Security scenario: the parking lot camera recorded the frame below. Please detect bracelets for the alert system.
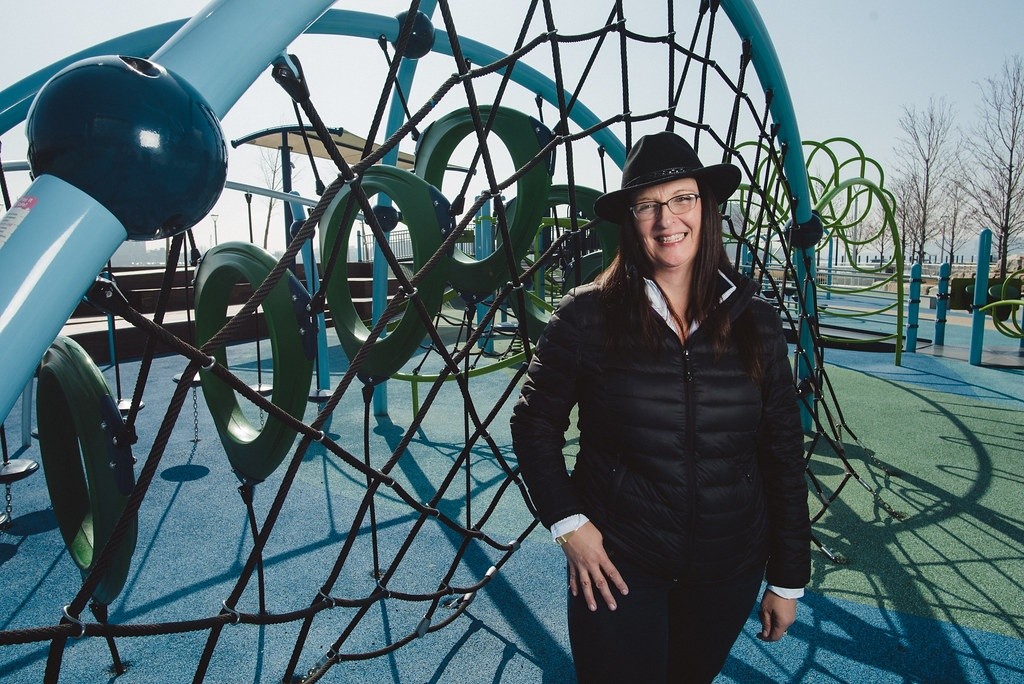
[556,531,576,546]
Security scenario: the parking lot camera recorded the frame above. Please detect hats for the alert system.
[592,131,742,225]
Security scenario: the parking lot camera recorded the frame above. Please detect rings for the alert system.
[783,630,787,634]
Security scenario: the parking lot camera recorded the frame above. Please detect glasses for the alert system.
[627,193,703,222]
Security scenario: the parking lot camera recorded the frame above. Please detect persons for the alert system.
[511,133,812,684]
